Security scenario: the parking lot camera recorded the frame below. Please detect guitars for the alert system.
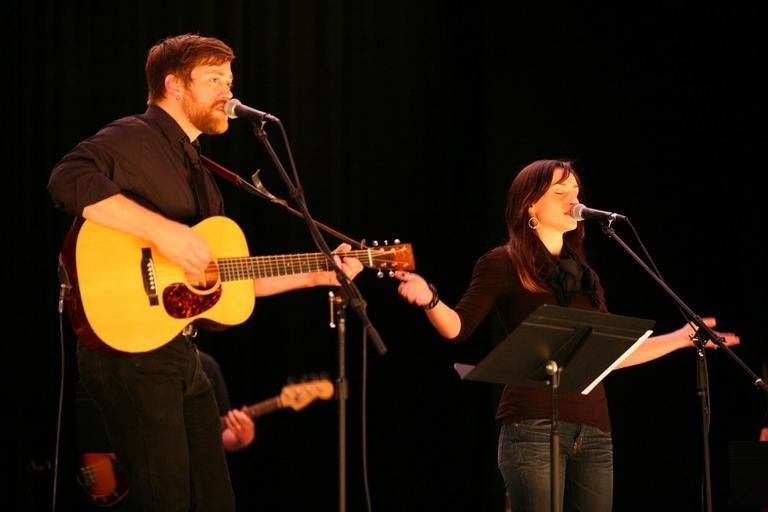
[59,215,416,358]
[66,372,335,509]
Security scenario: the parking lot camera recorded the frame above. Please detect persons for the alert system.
[394,159,739,512]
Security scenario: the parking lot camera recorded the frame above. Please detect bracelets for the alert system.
[417,283,439,310]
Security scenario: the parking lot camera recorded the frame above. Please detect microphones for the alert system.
[570,203,628,221]
[224,97,280,123]
